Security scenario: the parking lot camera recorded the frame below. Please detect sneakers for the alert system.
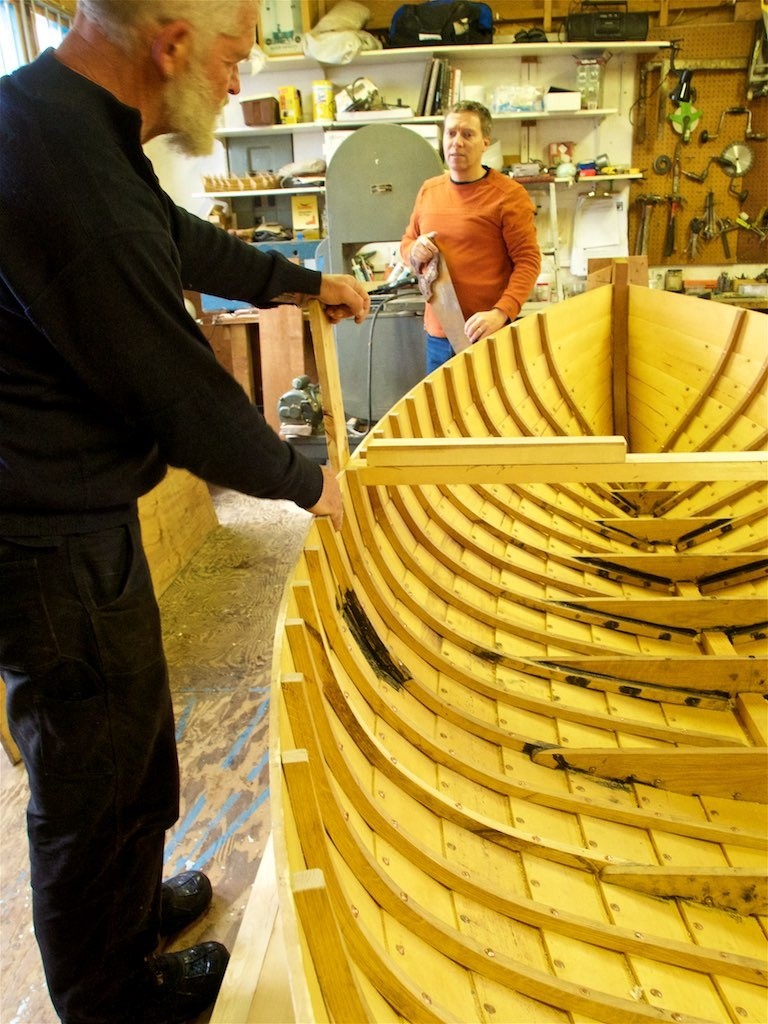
[158,869,213,941]
[119,941,231,1024]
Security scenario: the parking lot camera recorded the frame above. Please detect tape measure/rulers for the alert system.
[658,58,748,124]
[637,61,662,143]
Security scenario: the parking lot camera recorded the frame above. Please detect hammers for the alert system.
[664,194,687,257]
[632,194,660,256]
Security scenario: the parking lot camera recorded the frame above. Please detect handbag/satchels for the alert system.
[389,0,494,50]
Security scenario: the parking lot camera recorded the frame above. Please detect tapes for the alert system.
[577,159,595,169]
[597,154,609,167]
[654,155,671,174]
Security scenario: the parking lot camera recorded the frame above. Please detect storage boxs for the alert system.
[277,87,304,123]
[312,81,340,121]
[290,195,320,241]
[239,93,278,126]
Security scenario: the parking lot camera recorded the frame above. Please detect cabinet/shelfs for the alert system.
[204,38,672,321]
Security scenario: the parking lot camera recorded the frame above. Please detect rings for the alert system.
[477,328,484,334]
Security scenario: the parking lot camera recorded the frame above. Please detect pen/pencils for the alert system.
[368,289,384,295]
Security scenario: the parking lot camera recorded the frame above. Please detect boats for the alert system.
[267,257,767,1023]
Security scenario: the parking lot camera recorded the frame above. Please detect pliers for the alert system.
[671,143,682,179]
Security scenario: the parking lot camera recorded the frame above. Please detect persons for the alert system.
[400,99,542,374]
[0,0,369,1024]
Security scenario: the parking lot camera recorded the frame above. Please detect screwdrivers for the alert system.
[740,213,761,232]
[736,218,759,234]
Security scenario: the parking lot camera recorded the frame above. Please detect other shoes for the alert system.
[512,28,547,44]
